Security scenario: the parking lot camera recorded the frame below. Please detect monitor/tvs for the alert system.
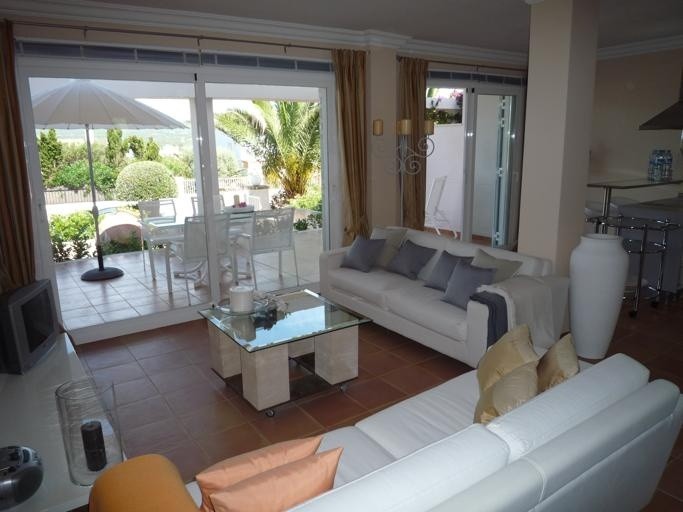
[0,279,58,375]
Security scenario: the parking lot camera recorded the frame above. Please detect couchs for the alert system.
[88,346,683,512]
[320,224,570,369]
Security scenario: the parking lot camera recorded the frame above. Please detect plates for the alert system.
[215,296,269,316]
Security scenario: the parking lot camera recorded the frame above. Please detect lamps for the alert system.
[373,118,435,225]
[636,66,683,142]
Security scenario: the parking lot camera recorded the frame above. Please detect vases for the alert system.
[568,230,629,360]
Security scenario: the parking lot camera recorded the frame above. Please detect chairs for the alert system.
[137,199,300,308]
[425,175,459,238]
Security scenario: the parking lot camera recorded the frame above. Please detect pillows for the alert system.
[339,233,522,311]
[470,325,580,425]
[194,436,322,511]
[209,446,344,511]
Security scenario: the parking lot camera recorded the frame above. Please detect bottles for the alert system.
[55,375,123,488]
[647,149,673,183]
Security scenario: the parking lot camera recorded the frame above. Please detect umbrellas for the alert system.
[30,80,190,280]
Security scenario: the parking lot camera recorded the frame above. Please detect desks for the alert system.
[1,332,127,512]
[588,215,681,319]
[587,178,683,234]
